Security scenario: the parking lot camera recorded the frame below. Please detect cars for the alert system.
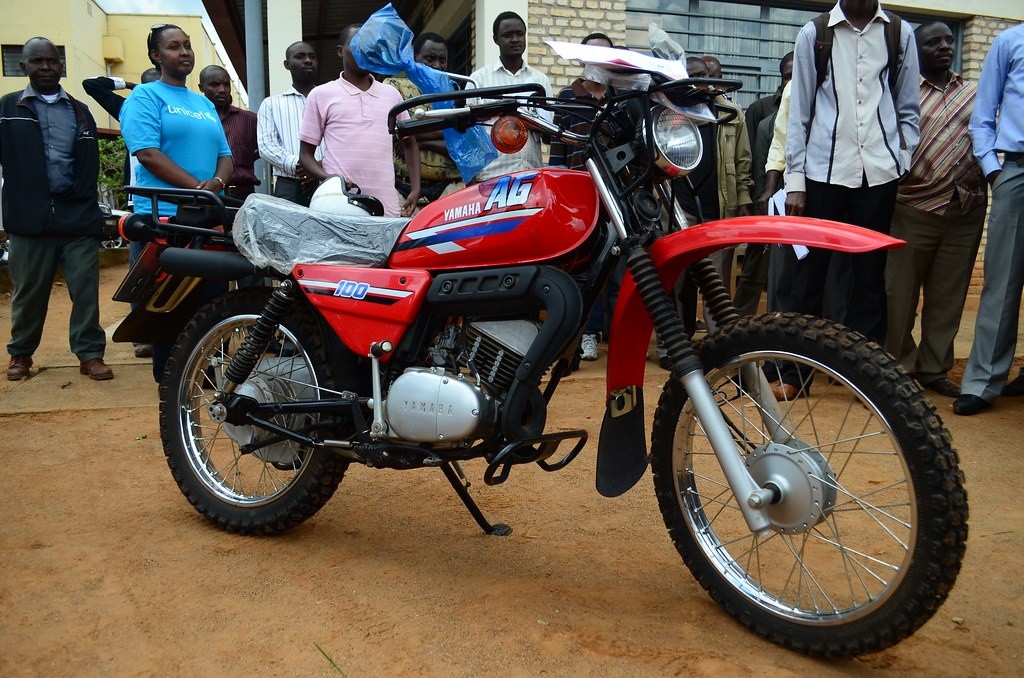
[99,201,133,249]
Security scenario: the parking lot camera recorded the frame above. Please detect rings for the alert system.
[304,176,307,179]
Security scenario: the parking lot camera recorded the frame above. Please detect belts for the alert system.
[1006,152,1024,161]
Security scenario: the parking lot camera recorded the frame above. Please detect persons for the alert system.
[465,12,554,182]
[257,24,465,225]
[549,33,651,362]
[684,0,1024,416]
[0,37,115,381]
[82,24,261,357]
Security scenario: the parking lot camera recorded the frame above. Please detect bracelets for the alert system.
[214,177,225,188]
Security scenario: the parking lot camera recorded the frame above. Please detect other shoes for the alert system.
[695,319,707,332]
[134,345,153,357]
[1000,375,1024,396]
[659,356,674,370]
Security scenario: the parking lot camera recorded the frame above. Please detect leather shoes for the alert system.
[771,381,810,401]
[953,394,996,415]
[922,378,961,397]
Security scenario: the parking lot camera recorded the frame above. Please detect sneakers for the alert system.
[579,334,598,360]
[80,358,113,380]
[7,356,33,380]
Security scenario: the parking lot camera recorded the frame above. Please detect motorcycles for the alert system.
[107,23,970,661]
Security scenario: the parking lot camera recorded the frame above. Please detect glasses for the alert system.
[150,24,178,45]
